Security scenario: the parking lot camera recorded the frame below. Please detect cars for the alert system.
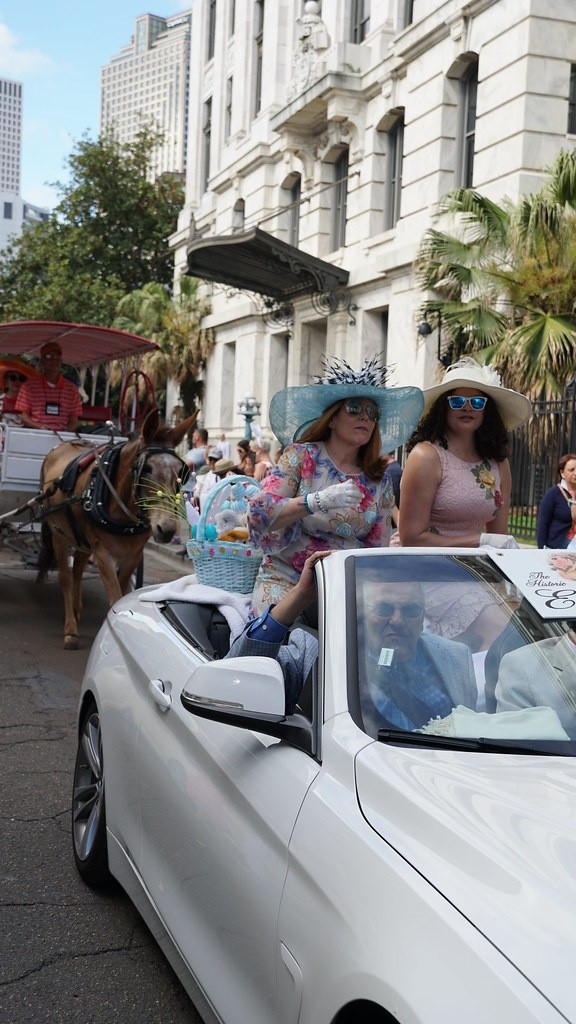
[72,549,576,1024]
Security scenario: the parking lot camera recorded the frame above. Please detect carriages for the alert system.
[0,321,201,651]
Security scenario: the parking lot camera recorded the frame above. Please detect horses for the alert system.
[35,403,200,651]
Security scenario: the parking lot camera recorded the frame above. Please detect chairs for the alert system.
[482,594,575,713]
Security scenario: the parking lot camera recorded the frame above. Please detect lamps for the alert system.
[418,309,454,373]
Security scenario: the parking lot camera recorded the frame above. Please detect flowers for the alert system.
[445,357,501,387]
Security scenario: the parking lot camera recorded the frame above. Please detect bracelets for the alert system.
[314,491,329,515]
[296,492,314,514]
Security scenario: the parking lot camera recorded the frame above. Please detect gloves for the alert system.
[480,533,521,549]
[304,478,365,514]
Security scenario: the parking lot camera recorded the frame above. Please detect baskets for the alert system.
[186,474,263,594]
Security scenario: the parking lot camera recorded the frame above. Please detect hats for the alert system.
[268,350,424,457]
[208,448,222,458]
[0,359,40,389]
[258,435,271,450]
[421,357,531,431]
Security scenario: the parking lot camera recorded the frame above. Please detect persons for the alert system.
[221,548,479,742]
[247,349,424,632]
[495,617,576,728]
[537,453,576,549]
[174,445,246,556]
[15,342,82,431]
[0,353,39,437]
[236,439,255,477]
[390,356,532,650]
[217,430,231,460]
[181,427,209,473]
[249,435,275,481]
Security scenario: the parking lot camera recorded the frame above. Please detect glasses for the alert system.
[366,602,423,618]
[238,449,242,452]
[447,396,488,410]
[44,354,62,359]
[9,374,27,383]
[345,400,381,422]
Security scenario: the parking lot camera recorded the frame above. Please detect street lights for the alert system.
[238,397,262,442]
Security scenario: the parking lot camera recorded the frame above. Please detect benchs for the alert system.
[0,397,112,449]
[164,600,229,657]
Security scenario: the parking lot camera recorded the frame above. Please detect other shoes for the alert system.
[175,550,187,556]
